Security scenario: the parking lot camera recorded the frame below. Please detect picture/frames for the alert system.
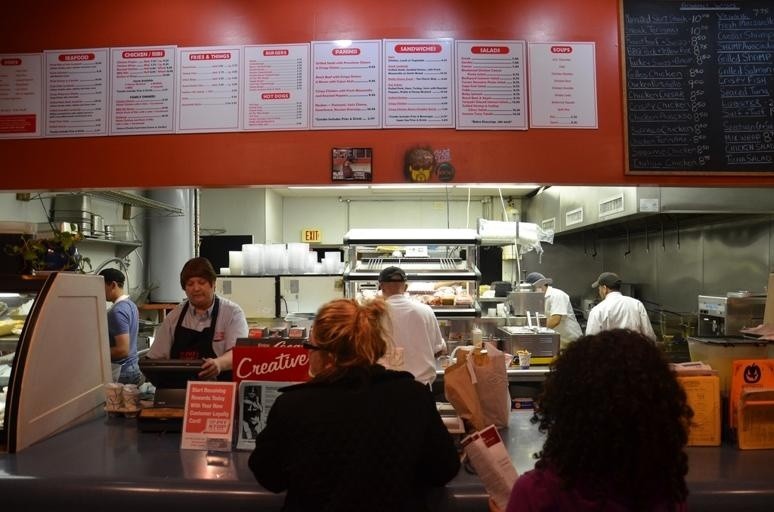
[331,148,372,181]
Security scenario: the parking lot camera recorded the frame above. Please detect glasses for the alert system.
[302,338,334,353]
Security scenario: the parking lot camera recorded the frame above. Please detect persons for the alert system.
[487,329,692,511]
[525,272,583,356]
[137,256,249,382]
[375,266,448,407]
[584,273,657,345]
[247,297,462,511]
[98,268,144,386]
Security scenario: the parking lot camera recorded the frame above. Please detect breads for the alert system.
[0,320,25,335]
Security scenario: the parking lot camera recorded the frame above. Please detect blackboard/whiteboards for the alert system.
[619,0,774,176]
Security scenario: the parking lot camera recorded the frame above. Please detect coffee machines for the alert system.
[697,294,767,338]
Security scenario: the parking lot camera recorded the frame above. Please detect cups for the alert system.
[496,304,504,316]
[518,352,530,368]
[487,308,496,317]
[105,383,122,409]
[663,335,673,352]
[122,384,139,408]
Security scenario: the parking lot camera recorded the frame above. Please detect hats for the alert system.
[99,268,125,283]
[180,257,216,291]
[591,272,622,289]
[526,272,546,284]
[378,266,407,290]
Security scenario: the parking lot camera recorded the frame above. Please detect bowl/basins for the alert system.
[227,243,341,275]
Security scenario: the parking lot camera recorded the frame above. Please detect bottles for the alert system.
[472,328,482,349]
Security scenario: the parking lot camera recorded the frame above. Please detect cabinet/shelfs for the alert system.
[343,227,482,318]
